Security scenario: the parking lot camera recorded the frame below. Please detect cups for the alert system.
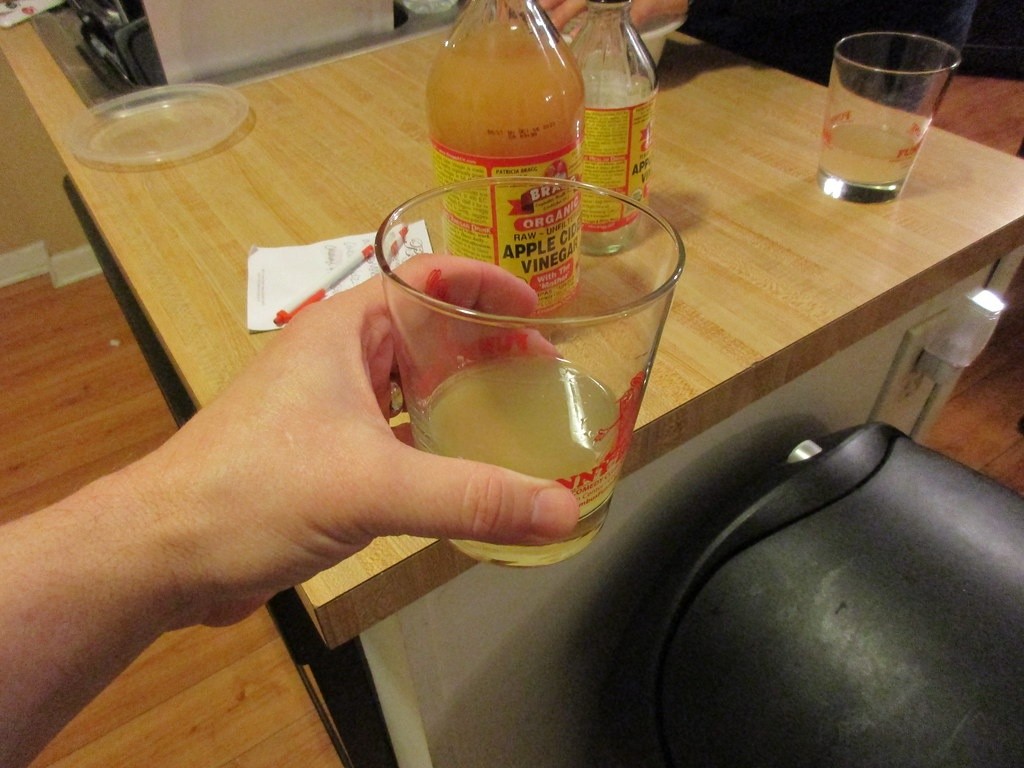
[816,31,962,205]
[374,173,685,569]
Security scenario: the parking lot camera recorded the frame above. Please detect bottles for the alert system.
[567,0,660,255]
[423,0,586,317]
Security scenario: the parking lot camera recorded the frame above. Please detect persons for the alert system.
[0,250,581,768]
[541,0,974,116]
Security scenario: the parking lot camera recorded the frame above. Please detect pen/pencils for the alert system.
[273,244,376,326]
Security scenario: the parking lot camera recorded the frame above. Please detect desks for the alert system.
[0,0,1023,767]
